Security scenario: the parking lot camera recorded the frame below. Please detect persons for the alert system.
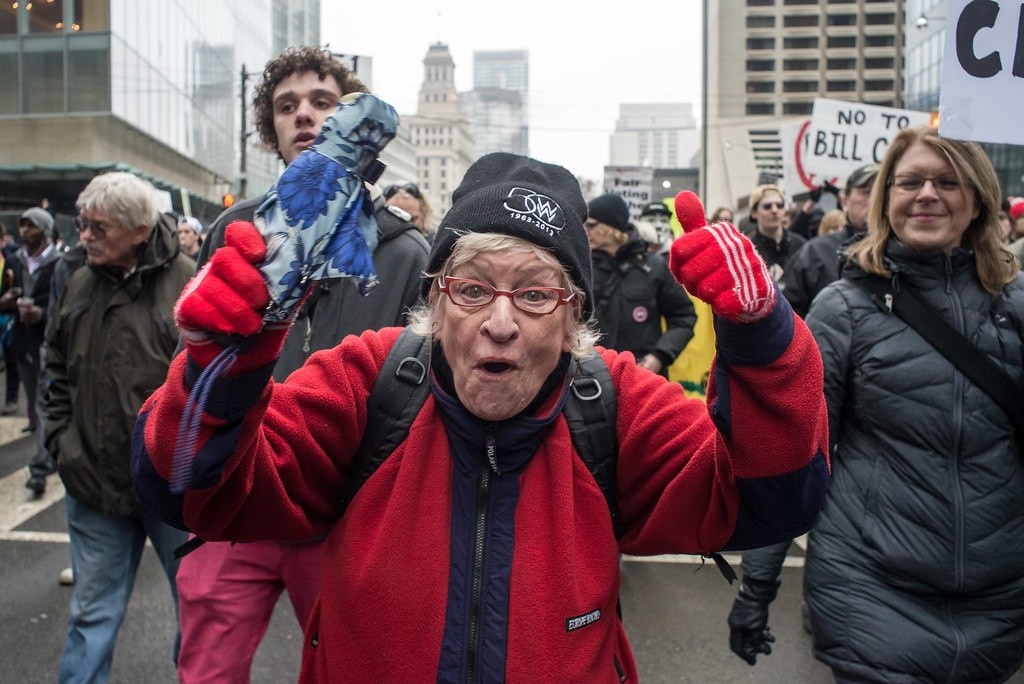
[0,207,88,492]
[782,180,846,240]
[42,198,54,217]
[43,171,197,684]
[709,207,733,224]
[178,216,204,261]
[583,194,697,380]
[130,152,832,684]
[746,184,807,270]
[384,183,435,245]
[173,46,432,684]
[777,165,879,320]
[996,195,1024,244]
[639,202,675,264]
[727,125,1024,684]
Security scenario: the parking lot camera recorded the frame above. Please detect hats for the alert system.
[748,184,786,223]
[19,207,54,239]
[844,165,879,196]
[588,194,633,232]
[639,203,673,220]
[421,153,595,325]
[176,214,205,247]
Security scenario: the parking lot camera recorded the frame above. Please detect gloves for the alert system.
[174,221,317,376]
[668,190,776,322]
[728,574,782,665]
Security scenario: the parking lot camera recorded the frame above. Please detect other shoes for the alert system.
[1,403,19,415]
[24,474,47,492]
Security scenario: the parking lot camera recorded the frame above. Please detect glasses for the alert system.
[585,220,600,230]
[756,200,784,210]
[382,183,424,202]
[887,175,973,192]
[438,276,576,314]
[75,217,123,239]
